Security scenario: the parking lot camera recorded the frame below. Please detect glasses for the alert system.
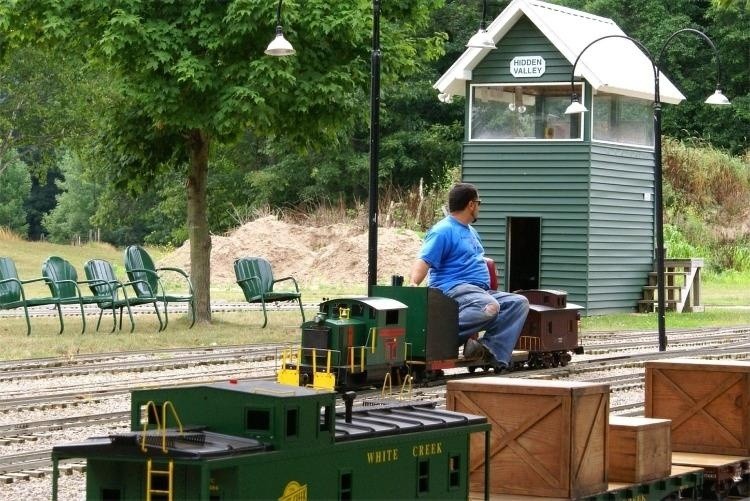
[472,200,481,206]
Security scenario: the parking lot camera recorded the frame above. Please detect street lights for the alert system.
[263,0,381,291]
[564,26,734,353]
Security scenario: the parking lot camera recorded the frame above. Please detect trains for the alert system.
[278,282,583,394]
[45,355,747,499]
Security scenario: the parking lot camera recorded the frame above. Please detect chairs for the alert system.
[234,256,305,328]
[0,245,196,336]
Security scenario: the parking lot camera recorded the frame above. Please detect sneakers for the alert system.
[462,337,491,361]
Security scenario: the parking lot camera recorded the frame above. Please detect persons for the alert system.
[406,184,529,368]
